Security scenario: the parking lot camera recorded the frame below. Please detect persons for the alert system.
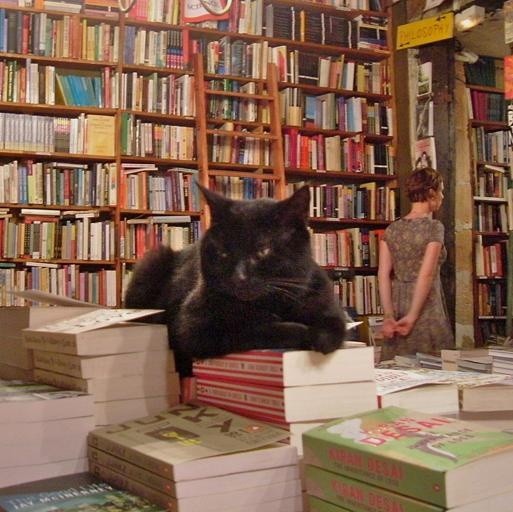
[378,169,447,357]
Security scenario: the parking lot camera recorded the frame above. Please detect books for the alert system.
[0,0,397,322]
[414,58,513,318]
[0,307,513,512]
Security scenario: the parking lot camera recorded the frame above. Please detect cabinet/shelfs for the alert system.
[0,0,405,327]
[462,54,512,348]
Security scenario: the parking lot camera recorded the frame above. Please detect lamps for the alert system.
[453,3,485,32]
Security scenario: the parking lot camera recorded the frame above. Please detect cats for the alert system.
[123,178,347,381]
